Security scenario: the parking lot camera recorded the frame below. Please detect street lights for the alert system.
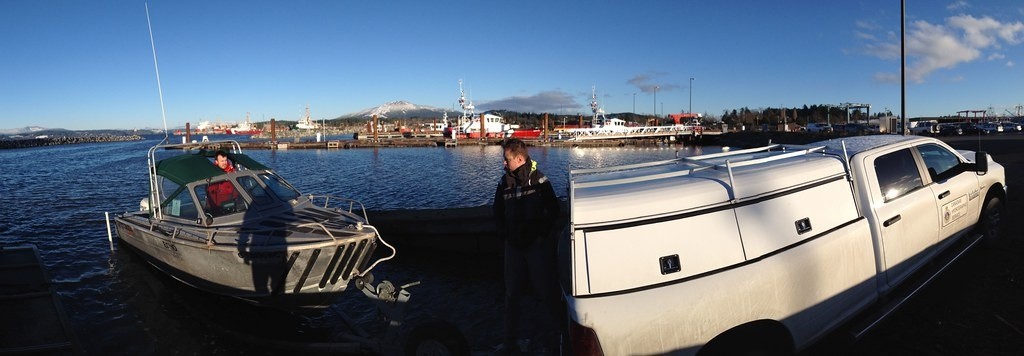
[654,86,659,126]
[633,93,637,127]
[690,78,695,126]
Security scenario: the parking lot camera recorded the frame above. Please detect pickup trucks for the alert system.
[567,135,1010,356]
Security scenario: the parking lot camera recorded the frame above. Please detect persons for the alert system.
[486,139,561,356]
[206,151,239,218]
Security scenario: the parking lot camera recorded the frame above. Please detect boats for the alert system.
[115,140,380,304]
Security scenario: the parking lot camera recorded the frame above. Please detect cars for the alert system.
[806,122,1022,135]
